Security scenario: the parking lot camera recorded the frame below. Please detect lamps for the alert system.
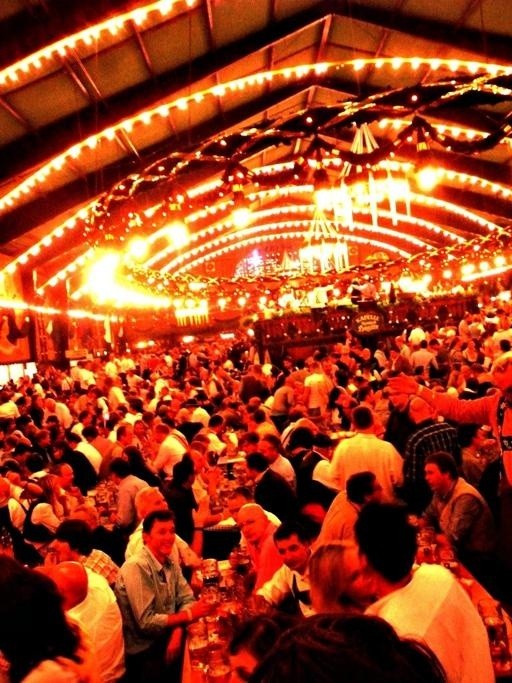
[299,206,348,274]
[341,125,411,229]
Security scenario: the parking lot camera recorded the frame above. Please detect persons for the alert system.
[0,272,512,683]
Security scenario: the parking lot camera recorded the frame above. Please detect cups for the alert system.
[415,527,512,601]
[187,559,236,683]
[96,482,120,525]
[212,470,246,515]
